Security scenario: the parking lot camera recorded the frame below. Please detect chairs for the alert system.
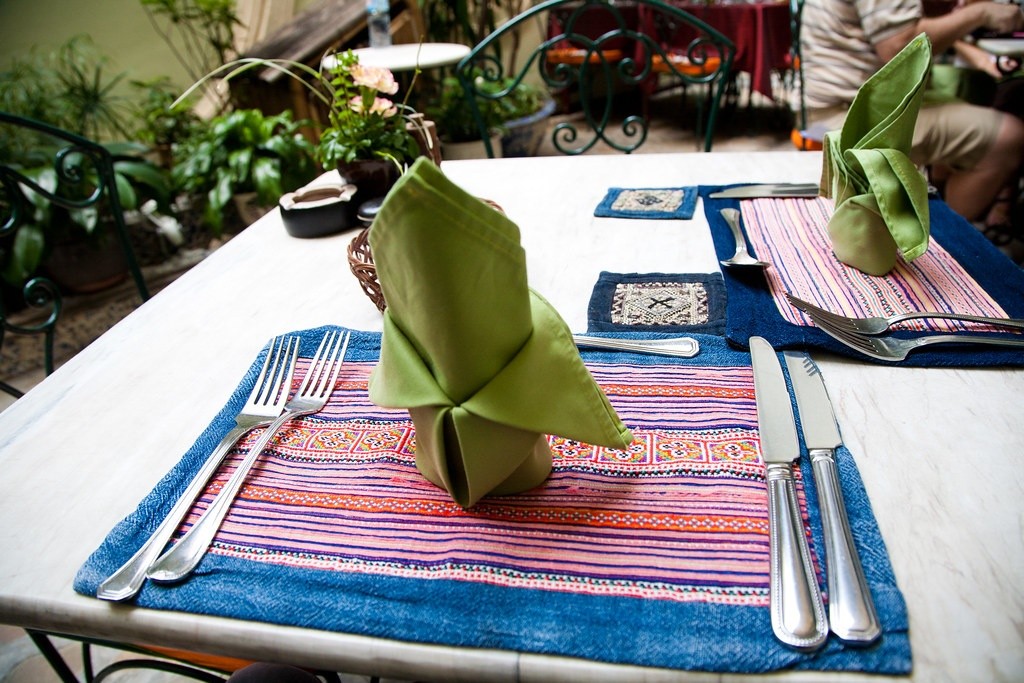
[790,0,828,151]
[452,0,739,159]
[1,111,344,683]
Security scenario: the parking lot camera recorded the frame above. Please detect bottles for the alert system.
[366,0,391,50]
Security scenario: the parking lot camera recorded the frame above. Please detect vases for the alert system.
[336,145,401,195]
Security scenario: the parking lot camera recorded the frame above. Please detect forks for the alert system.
[144,330,354,582]
[783,290,1024,332]
[95,332,300,602]
[804,306,1024,363]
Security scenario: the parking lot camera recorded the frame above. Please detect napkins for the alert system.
[369,155,634,508]
[819,31,934,277]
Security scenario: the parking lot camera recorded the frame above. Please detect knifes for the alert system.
[782,350,882,647]
[709,183,821,199]
[750,334,828,648]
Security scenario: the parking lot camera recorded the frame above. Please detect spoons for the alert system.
[719,207,772,272]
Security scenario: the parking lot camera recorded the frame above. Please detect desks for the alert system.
[549,0,793,109]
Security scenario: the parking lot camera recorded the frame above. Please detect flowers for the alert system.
[169,47,432,177]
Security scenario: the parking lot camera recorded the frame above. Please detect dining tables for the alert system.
[978,38,1024,57]
[324,43,475,75]
[0,149,1024,683]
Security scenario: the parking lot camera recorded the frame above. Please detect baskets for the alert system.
[346,196,507,316]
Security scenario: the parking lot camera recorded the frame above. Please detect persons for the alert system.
[800,0,1024,234]
